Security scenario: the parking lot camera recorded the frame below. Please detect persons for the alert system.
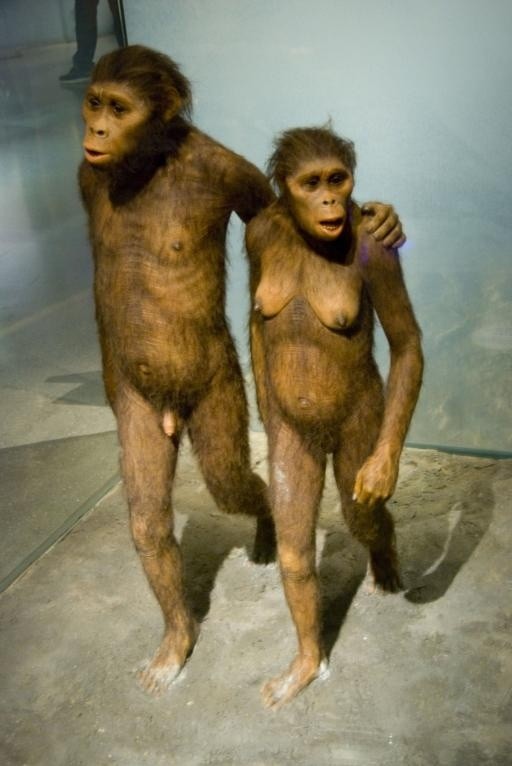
[59,2,124,83]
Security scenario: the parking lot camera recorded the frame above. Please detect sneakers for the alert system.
[58,65,93,84]
